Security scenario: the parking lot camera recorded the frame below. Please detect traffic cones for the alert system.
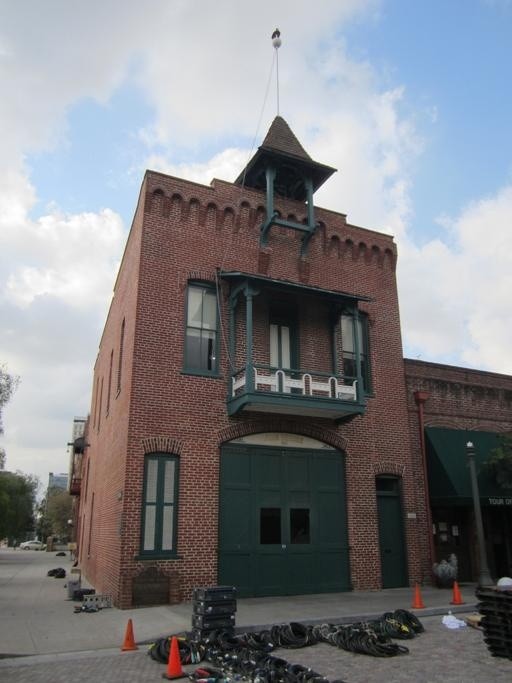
[448,579,467,605]
[119,616,144,651]
[161,634,189,681]
[409,581,426,609]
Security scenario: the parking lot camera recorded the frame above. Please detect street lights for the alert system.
[463,438,499,602]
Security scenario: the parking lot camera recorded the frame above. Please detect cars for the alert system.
[19,539,47,551]
[54,535,71,545]
